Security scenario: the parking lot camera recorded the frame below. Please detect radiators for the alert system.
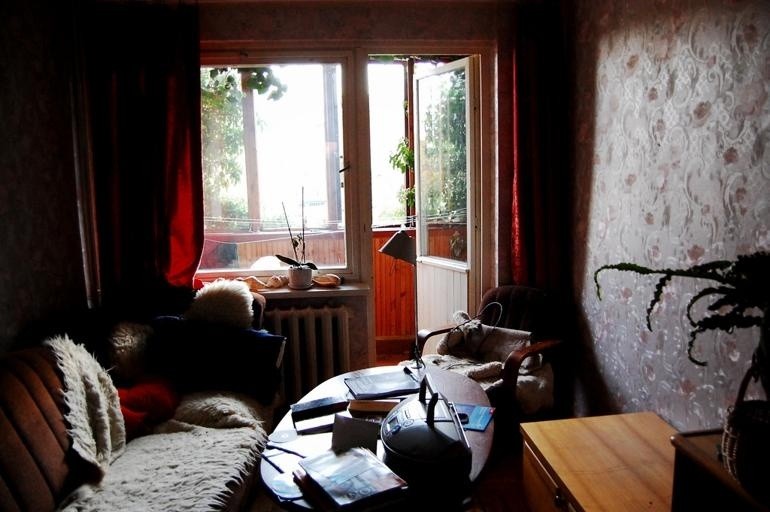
[264,307,353,405]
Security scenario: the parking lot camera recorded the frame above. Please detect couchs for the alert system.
[0,316,287,512]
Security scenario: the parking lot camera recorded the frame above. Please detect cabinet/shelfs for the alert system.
[520,411,770,512]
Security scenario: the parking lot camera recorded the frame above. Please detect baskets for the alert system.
[721,353,769,497]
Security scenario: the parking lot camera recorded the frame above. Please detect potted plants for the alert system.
[276,233,317,290]
[594,251,770,496]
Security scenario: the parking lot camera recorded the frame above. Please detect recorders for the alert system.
[379,373,473,484]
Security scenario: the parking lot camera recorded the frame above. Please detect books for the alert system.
[290,369,496,512]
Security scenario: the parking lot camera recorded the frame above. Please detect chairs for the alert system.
[420,285,576,452]
